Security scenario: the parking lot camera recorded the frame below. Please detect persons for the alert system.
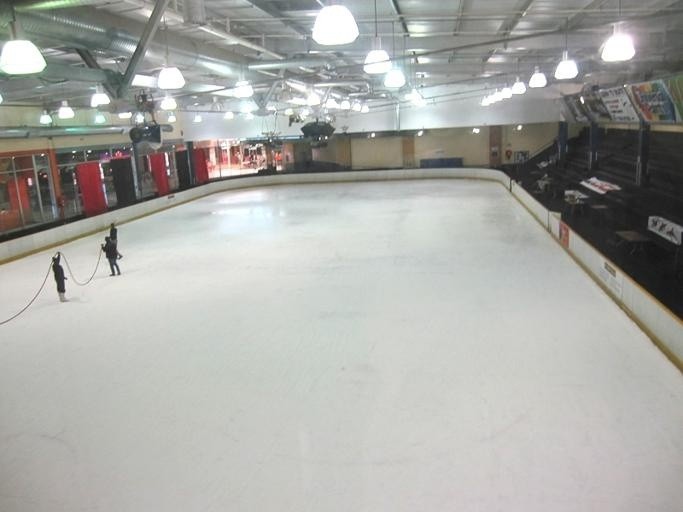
[100,236,121,277]
[51,251,69,302]
[108,222,123,259]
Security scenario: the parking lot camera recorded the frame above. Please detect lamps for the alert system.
[527,48,548,89]
[86,93,374,127]
[0,1,47,76]
[553,17,578,80]
[599,3,636,63]
[56,80,76,121]
[382,20,405,90]
[363,1,392,75]
[480,58,527,107]
[405,51,427,112]
[154,3,186,91]
[39,97,54,126]
[312,3,361,48]
